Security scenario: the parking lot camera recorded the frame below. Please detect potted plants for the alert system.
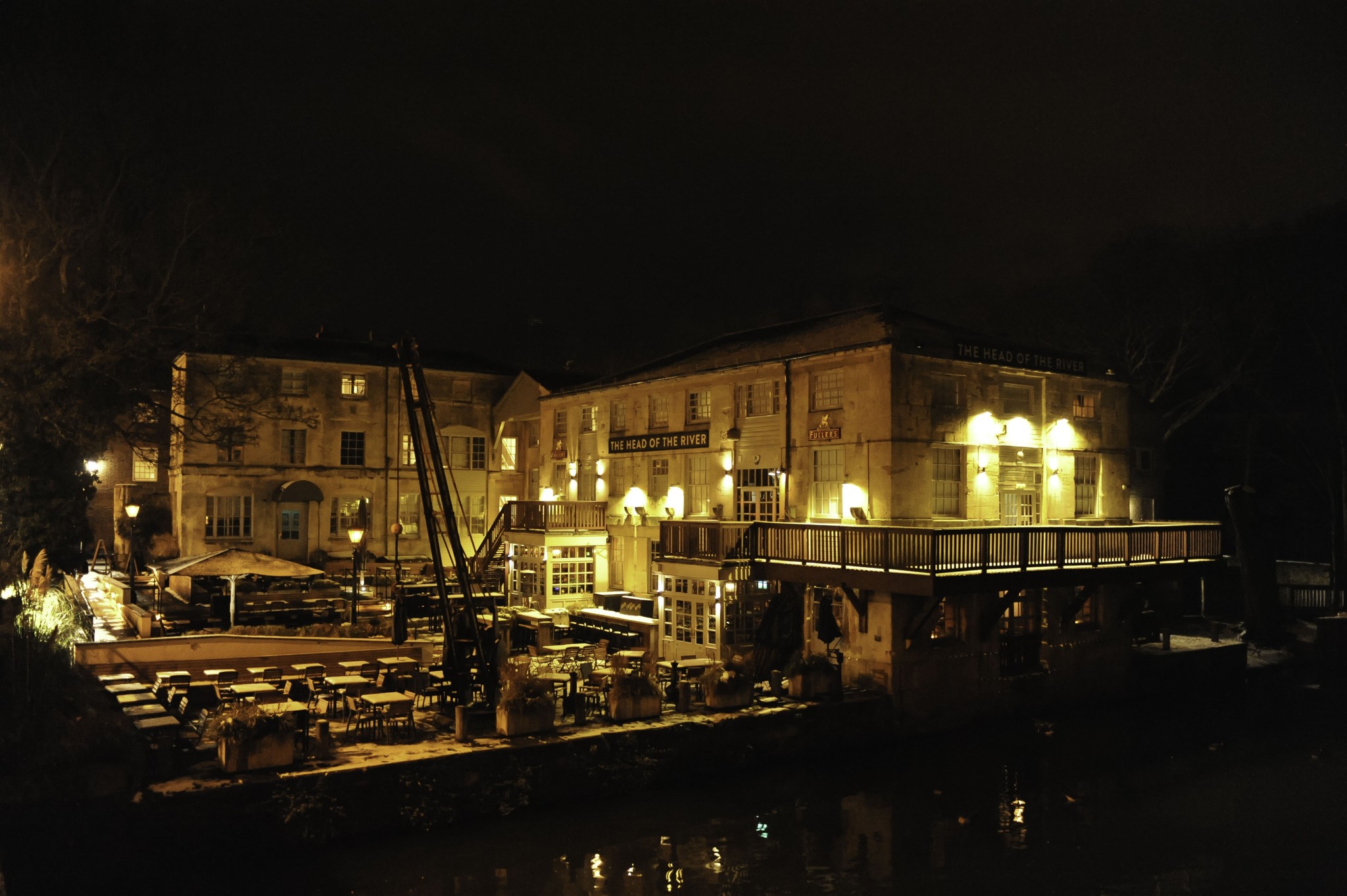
[787,651,840,697]
[699,661,755,707]
[209,698,298,773]
[608,652,663,720]
[496,661,556,735]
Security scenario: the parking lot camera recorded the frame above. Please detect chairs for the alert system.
[513,614,703,709]
[218,668,310,751]
[152,675,208,757]
[252,601,285,625]
[299,661,485,745]
[313,599,346,624]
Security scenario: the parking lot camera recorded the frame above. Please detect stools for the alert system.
[555,625,569,643]
[517,623,537,652]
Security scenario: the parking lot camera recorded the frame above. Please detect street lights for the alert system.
[124,497,140,604]
[347,522,364,625]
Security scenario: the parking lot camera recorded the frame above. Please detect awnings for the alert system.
[272,480,324,501]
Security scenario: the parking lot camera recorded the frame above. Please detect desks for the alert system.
[203,667,308,714]
[396,583,507,631]
[291,657,418,741]
[429,669,478,712]
[378,567,410,576]
[515,613,553,630]
[98,671,191,757]
[534,643,723,700]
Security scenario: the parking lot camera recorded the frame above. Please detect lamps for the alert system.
[624,505,676,517]
[850,507,866,520]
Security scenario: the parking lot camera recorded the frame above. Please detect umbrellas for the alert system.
[356,496,368,572]
[145,547,326,627]
[392,585,407,660]
